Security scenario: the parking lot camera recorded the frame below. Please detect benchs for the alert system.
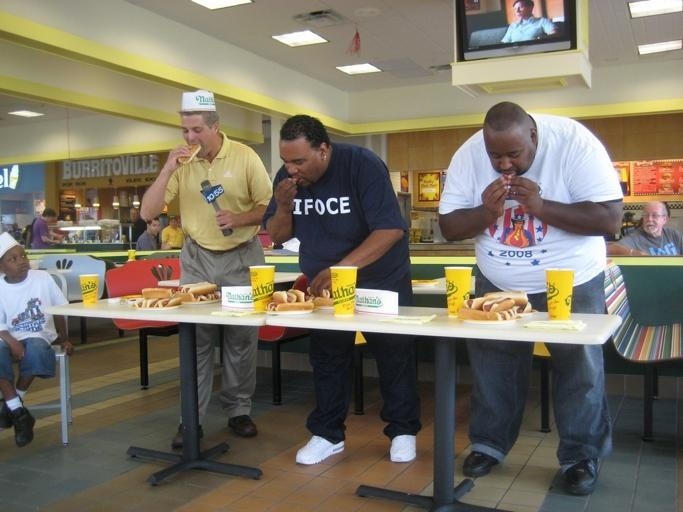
[354,274,555,434]
[107,258,321,405]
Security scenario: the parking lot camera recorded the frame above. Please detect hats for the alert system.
[0,229,20,259]
[180,88,218,113]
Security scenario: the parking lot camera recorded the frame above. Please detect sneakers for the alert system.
[565,455,600,497]
[0,397,37,449]
[170,424,205,450]
[225,414,258,438]
[389,433,417,463]
[295,434,345,466]
[462,449,500,478]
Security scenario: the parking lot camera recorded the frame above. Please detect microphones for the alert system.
[199,179,234,237]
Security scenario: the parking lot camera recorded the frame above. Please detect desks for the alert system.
[38,282,625,510]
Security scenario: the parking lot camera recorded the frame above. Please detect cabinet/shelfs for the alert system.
[45,219,126,248]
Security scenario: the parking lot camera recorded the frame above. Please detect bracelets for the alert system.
[629,249,635,256]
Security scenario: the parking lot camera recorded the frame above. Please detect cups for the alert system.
[545,270,575,321]
[248,265,275,314]
[128,249,136,260]
[79,273,99,307]
[329,265,360,317]
[443,266,473,320]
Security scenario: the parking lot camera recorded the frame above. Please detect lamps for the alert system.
[110,188,120,211]
[72,198,81,208]
[131,185,142,210]
[91,188,100,207]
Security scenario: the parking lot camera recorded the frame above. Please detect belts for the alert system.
[188,236,257,254]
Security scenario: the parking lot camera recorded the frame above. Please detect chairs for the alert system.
[41,255,124,343]
[13,272,73,444]
[603,263,683,440]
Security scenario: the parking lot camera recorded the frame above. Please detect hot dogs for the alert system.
[133,281,221,309]
[177,144,201,164]
[265,286,330,312]
[458,290,532,322]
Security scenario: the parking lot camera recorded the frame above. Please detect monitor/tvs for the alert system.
[455,0,579,62]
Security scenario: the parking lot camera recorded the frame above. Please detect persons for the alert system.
[500,0,562,46]
[22,219,38,248]
[263,116,422,465]
[127,209,184,254]
[139,90,273,447]
[436,102,623,497]
[606,201,682,262]
[34,208,63,249]
[0,231,74,448]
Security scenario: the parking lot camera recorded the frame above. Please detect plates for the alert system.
[463,310,539,325]
[263,308,314,315]
[134,300,183,311]
[313,305,335,311]
[178,298,222,305]
